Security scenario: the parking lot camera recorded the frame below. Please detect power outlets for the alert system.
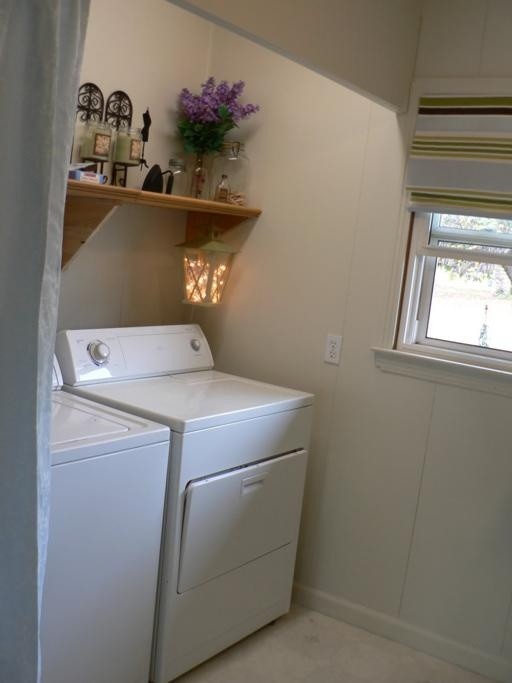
[324,333,342,365]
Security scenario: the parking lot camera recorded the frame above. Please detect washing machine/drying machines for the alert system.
[55,323,317,683]
[39,355,171,683]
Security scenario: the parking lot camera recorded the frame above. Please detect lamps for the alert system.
[176,224,237,308]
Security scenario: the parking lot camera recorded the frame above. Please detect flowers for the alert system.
[180,76,260,131]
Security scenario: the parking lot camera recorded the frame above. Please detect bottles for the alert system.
[214,173,232,203]
[80,119,113,160]
[115,125,143,159]
[208,140,249,208]
[163,158,188,195]
